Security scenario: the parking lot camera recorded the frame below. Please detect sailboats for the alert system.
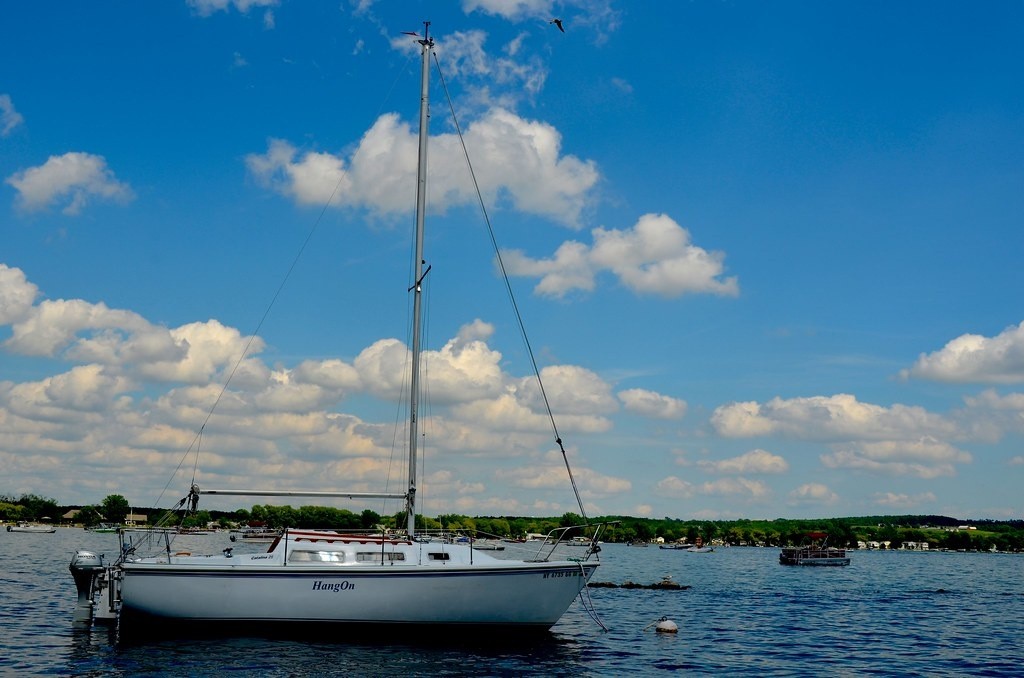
[70,18,603,629]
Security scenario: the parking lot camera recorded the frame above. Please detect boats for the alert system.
[780,532,852,567]
[740,540,747,548]
[234,539,275,543]
[509,535,527,544]
[526,533,554,545]
[83,523,122,534]
[467,537,505,551]
[242,528,280,539]
[659,539,693,550]
[649,575,689,590]
[566,536,604,546]
[7,520,56,533]
[687,545,713,553]
[626,541,648,547]
